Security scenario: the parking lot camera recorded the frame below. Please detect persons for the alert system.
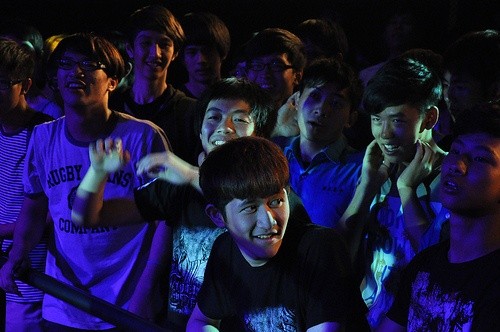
[108,5,200,165]
[0,36,56,332]
[246,28,307,107]
[298,9,449,149]
[0,22,71,120]
[71,77,312,332]
[375,101,500,332]
[187,136,370,332]
[0,32,173,332]
[335,55,451,332]
[171,13,229,100]
[437,30,500,153]
[284,58,366,229]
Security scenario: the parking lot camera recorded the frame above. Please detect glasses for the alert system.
[248,60,297,72]
[56,57,107,71]
[0,76,22,90]
